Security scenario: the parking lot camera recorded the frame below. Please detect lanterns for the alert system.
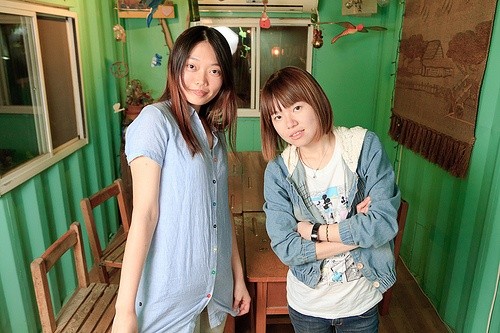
[272,44,287,60]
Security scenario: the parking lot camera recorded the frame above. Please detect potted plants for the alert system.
[126,80,151,113]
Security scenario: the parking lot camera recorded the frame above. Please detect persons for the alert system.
[110,25,251,333]
[259,66,402,333]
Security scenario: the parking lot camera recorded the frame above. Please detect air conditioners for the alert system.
[198,1,318,14]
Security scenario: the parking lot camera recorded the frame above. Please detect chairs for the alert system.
[81,178,130,283]
[31,221,120,332]
[380,196,409,315]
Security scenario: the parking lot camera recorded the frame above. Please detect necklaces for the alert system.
[301,134,331,179]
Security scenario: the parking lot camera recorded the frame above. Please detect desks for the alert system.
[223,152,290,332]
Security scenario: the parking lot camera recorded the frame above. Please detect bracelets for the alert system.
[325,223,329,241]
[310,222,322,243]
